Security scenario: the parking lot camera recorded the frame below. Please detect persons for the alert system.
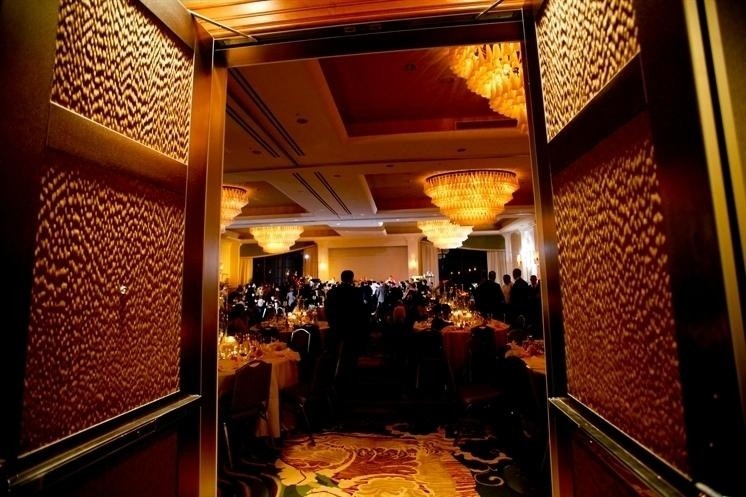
[225,269,542,389]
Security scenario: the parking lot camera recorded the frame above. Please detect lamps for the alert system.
[420,170,520,226]
[416,220,474,249]
[219,185,248,233]
[249,223,305,254]
[450,41,529,135]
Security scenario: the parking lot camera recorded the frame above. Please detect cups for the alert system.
[220,332,268,362]
